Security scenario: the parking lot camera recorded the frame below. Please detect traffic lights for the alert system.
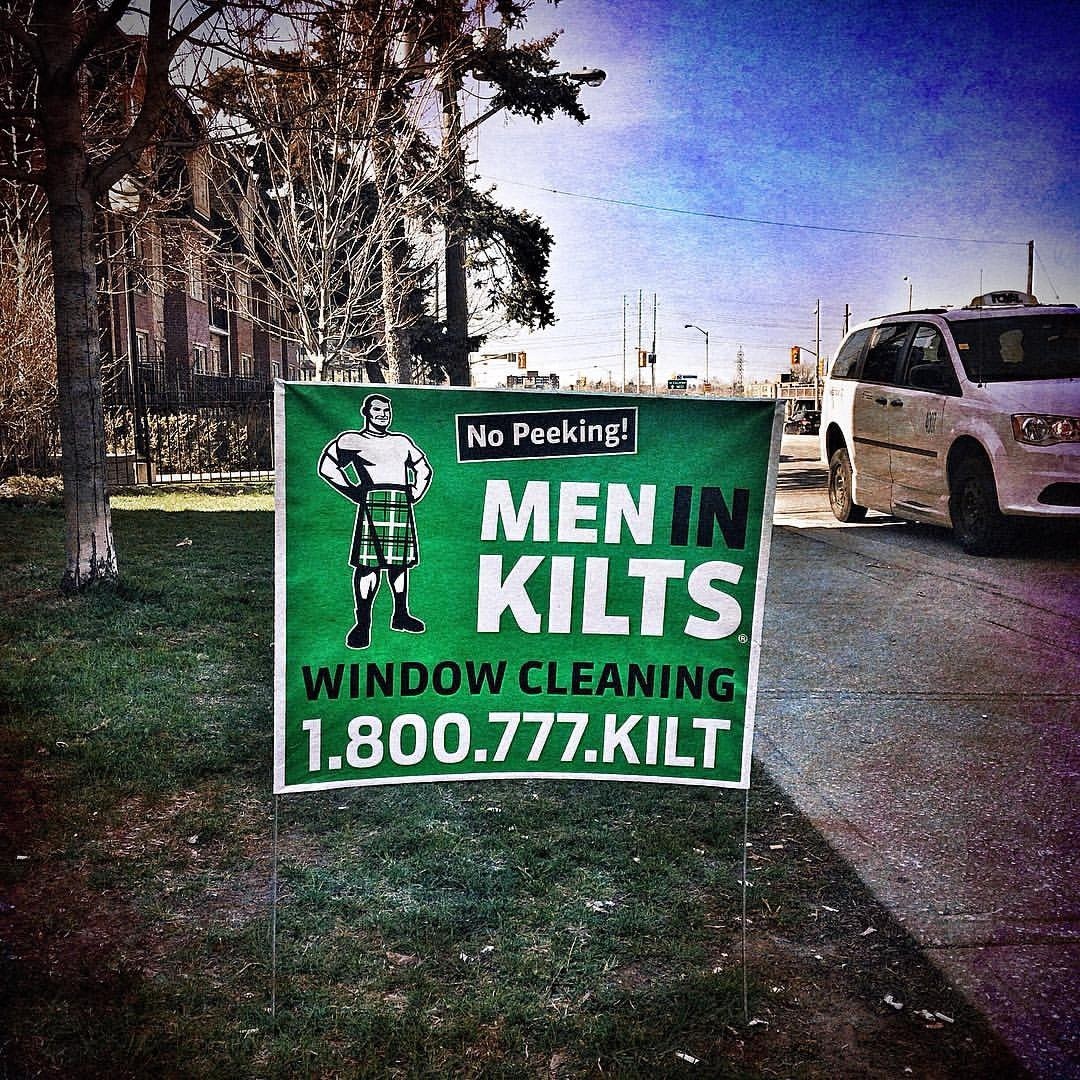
[792,348,802,366]
[818,358,824,376]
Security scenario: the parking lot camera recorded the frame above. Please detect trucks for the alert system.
[772,382,825,435]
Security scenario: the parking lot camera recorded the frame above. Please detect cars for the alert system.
[815,290,1080,558]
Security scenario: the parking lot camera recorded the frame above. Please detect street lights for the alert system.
[594,366,613,394]
[685,324,710,399]
